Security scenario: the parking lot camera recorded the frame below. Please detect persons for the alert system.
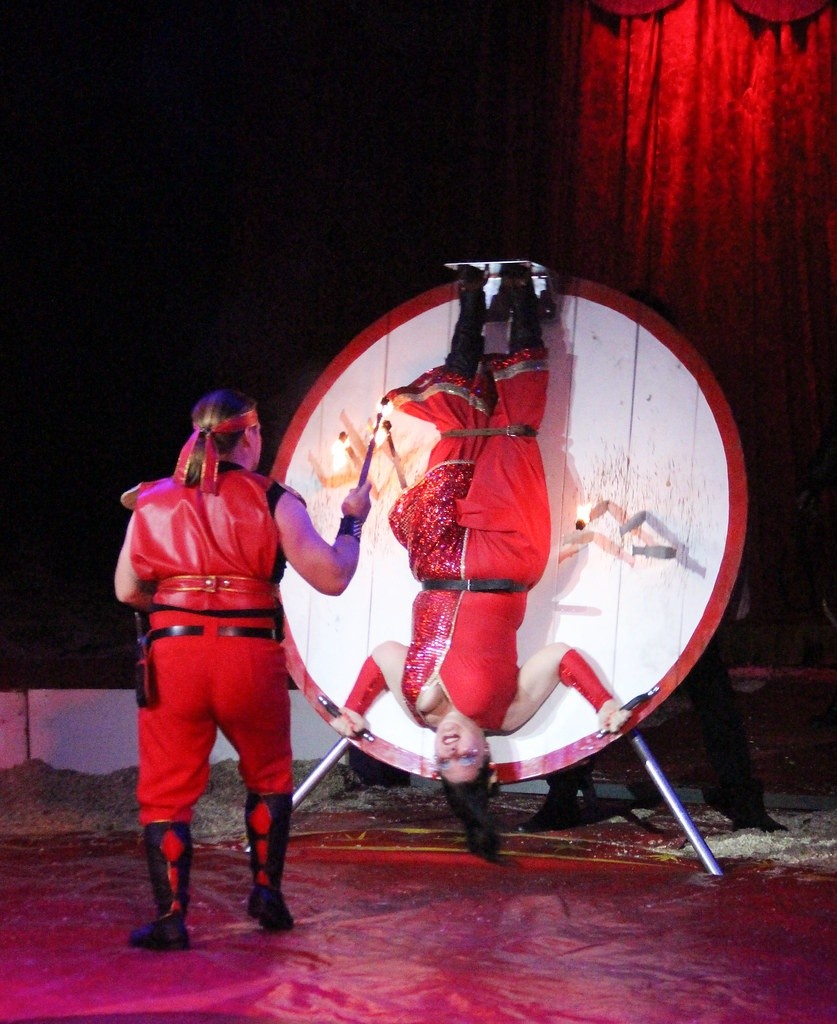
[329,264,633,810]
[115,388,373,950]
[795,361,837,731]
[515,287,788,834]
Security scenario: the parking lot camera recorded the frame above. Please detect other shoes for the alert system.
[129,923,191,952]
[247,888,293,930]
[809,705,837,728]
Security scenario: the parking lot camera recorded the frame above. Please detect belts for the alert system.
[423,578,525,592]
[145,625,281,640]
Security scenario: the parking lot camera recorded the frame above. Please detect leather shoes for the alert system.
[514,789,598,832]
[733,814,789,834]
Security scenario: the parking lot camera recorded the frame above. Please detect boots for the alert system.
[497,266,542,357]
[443,265,488,379]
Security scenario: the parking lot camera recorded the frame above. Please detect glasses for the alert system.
[438,753,477,770]
